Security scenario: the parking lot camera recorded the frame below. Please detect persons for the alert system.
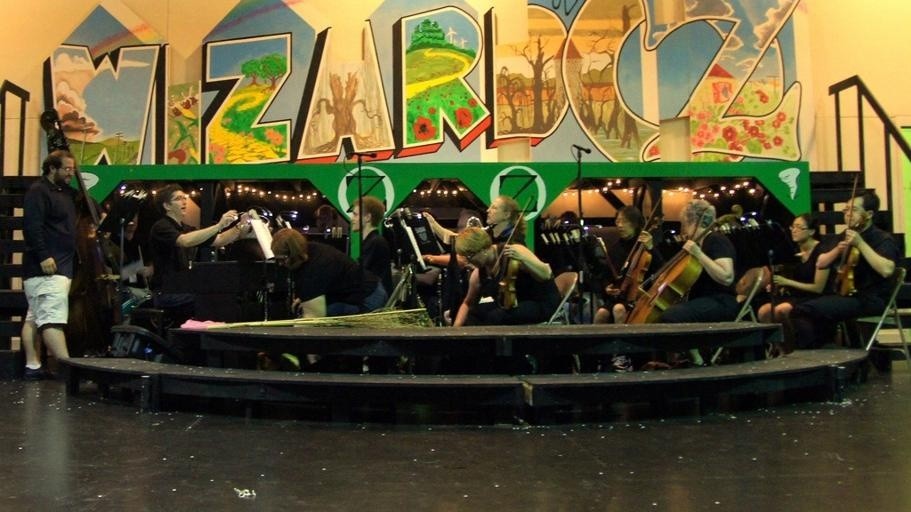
[20,151,78,379]
[592,186,903,353]
[149,186,562,327]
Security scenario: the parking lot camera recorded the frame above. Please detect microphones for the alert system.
[573,144,590,153]
[349,152,376,157]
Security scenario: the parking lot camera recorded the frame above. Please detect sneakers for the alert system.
[611,354,633,373]
[22,366,53,379]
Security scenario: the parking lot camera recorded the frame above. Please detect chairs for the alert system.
[855,266,910,359]
[543,270,578,325]
[708,268,770,365]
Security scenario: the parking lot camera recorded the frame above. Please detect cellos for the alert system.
[626,204,744,325]
[40,108,122,337]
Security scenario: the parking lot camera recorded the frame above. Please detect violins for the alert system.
[497,214,519,309]
[832,212,868,297]
[626,216,662,303]
